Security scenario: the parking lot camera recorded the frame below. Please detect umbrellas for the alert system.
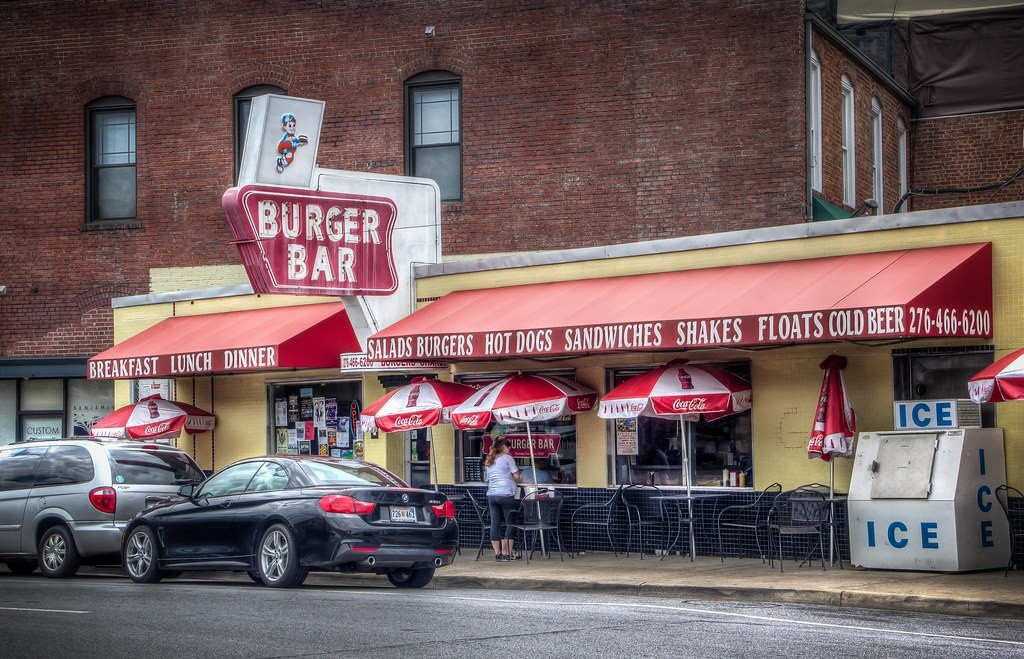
[452,371,599,556]
[968,348,1024,404]
[360,377,485,490]
[808,355,855,566]
[596,359,753,560]
[89,396,216,443]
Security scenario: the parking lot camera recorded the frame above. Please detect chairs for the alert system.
[448,482,842,572]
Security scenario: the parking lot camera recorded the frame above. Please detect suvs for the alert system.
[0,437,211,580]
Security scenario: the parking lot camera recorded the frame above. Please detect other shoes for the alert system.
[495,552,503,560]
[502,553,524,560]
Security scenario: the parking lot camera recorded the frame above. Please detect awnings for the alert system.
[86,301,362,379]
[365,241,994,362]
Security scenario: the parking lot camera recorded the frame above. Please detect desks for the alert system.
[787,496,846,571]
[648,494,731,559]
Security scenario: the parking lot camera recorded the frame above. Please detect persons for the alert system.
[521,458,554,483]
[485,439,522,561]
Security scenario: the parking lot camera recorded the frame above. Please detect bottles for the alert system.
[723,440,749,487]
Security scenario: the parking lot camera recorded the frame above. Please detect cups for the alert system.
[548,485,556,498]
[514,485,537,499]
[645,473,654,486]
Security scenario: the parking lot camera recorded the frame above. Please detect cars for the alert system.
[120,454,459,588]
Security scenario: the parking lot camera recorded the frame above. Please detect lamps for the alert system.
[424,26,435,38]
